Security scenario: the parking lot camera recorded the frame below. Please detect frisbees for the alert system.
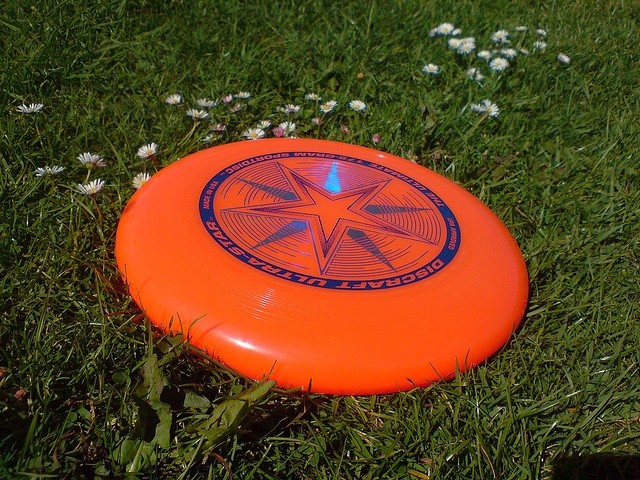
[115,138,529,396]
